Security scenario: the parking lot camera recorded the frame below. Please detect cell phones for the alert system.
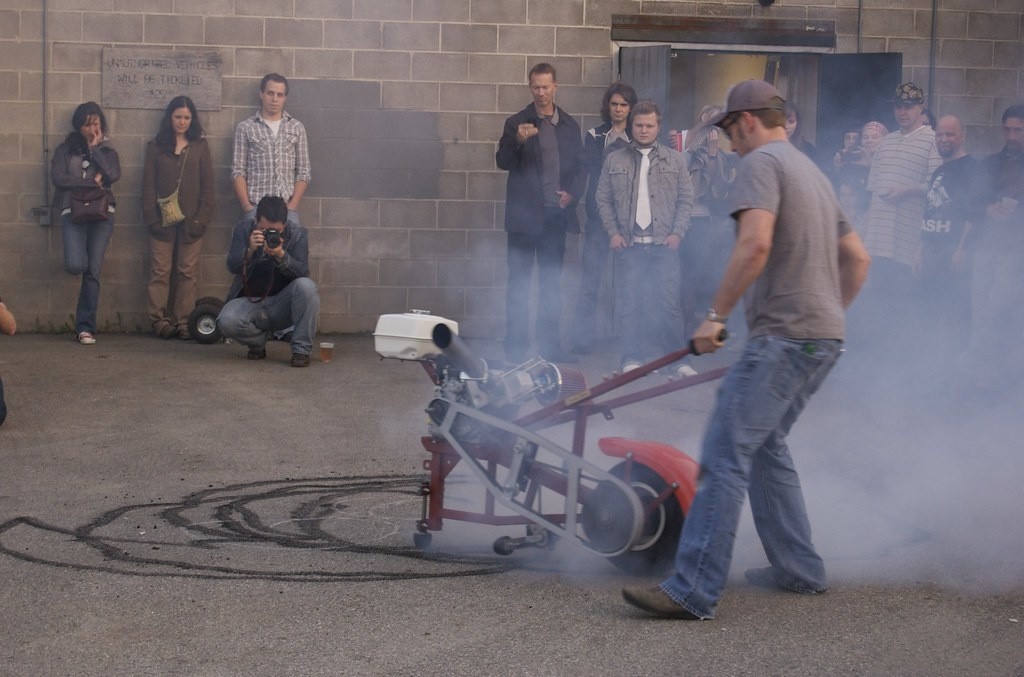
[527,116,537,128]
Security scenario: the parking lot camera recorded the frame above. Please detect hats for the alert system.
[704,79,785,126]
[888,83,924,104]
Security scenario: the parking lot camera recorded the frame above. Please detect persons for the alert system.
[144,95,214,340]
[0,298,17,426]
[621,76,869,619]
[496,63,587,365]
[569,83,1024,408]
[51,102,121,344]
[217,194,321,367]
[232,73,311,341]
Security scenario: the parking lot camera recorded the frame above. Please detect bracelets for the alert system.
[242,201,249,209]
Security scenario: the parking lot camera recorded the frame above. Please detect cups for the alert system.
[320,342,334,362]
[1003,197,1017,214]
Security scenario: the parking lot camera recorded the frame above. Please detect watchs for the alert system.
[707,307,726,324]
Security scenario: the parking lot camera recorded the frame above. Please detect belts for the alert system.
[633,236,653,244]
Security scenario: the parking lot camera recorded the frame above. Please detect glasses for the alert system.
[722,111,755,140]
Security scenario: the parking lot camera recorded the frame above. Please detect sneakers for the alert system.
[160,324,176,340]
[291,353,309,367]
[621,585,699,620]
[176,322,194,340]
[78,331,96,344]
[744,565,796,592]
[248,347,266,359]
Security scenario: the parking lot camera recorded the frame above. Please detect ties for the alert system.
[636,148,653,231]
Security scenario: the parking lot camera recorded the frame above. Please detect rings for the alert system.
[525,129,529,135]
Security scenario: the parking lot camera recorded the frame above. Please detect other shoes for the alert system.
[668,365,697,379]
[507,350,531,363]
[540,349,578,363]
[282,331,295,341]
[622,364,639,374]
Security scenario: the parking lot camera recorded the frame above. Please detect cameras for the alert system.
[261,227,284,249]
[841,147,864,161]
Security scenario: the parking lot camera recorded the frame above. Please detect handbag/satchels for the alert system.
[157,189,186,228]
[70,188,109,222]
